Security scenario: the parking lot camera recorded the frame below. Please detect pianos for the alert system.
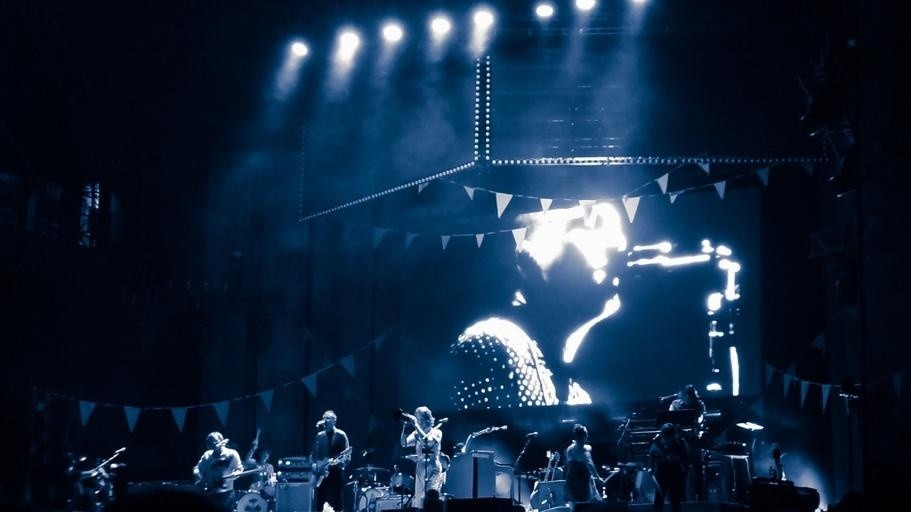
[441,451,515,499]
[625,427,703,446]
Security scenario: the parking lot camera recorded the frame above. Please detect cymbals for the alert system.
[356,468,389,473]
[401,455,418,463]
[737,422,764,430]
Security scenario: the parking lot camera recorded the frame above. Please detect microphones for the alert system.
[562,419,575,423]
[664,393,678,399]
[547,488,554,505]
[528,432,538,436]
[317,419,326,425]
[438,417,449,422]
[628,239,672,256]
[216,438,229,447]
[115,447,126,453]
[492,425,508,431]
[256,504,261,511]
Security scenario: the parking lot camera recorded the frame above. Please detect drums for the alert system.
[390,474,415,493]
[719,455,749,502]
[349,481,390,512]
[230,489,270,512]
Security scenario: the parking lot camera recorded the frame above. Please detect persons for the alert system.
[441,201,633,414]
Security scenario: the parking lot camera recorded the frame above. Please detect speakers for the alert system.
[475,450,496,498]
[275,482,314,512]
[531,479,567,512]
[440,454,474,499]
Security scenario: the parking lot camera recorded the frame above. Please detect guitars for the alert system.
[310,447,352,488]
[263,472,278,496]
[197,464,267,489]
[530,451,554,509]
